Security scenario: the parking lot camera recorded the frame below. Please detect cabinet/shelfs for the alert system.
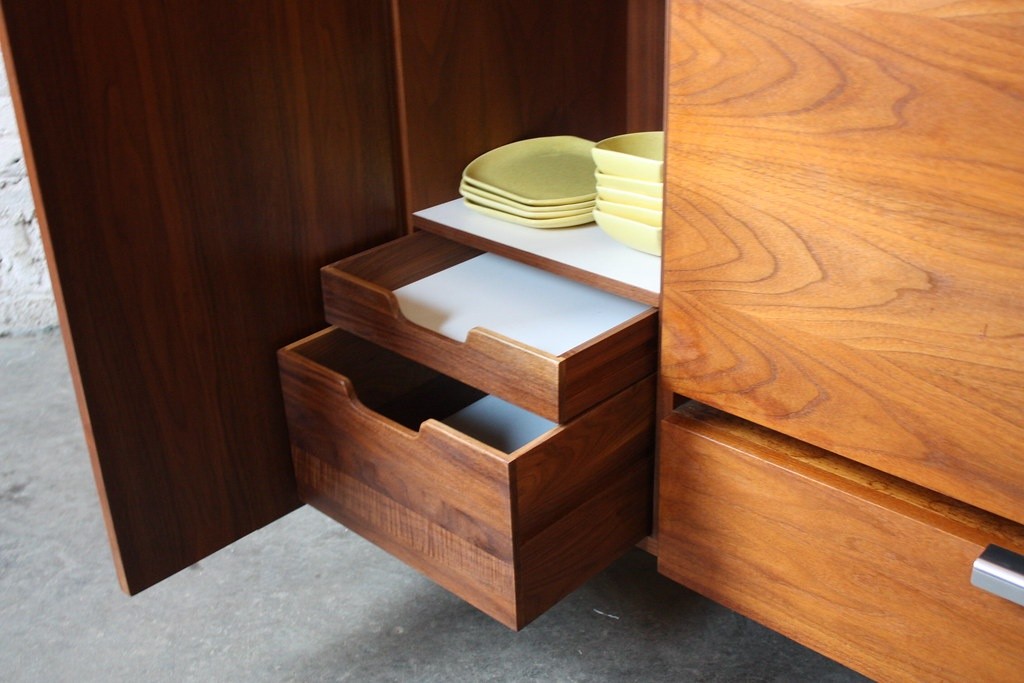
[0,0,1024,683]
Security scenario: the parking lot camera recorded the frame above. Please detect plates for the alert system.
[591,132,664,258]
[459,134,596,228]
